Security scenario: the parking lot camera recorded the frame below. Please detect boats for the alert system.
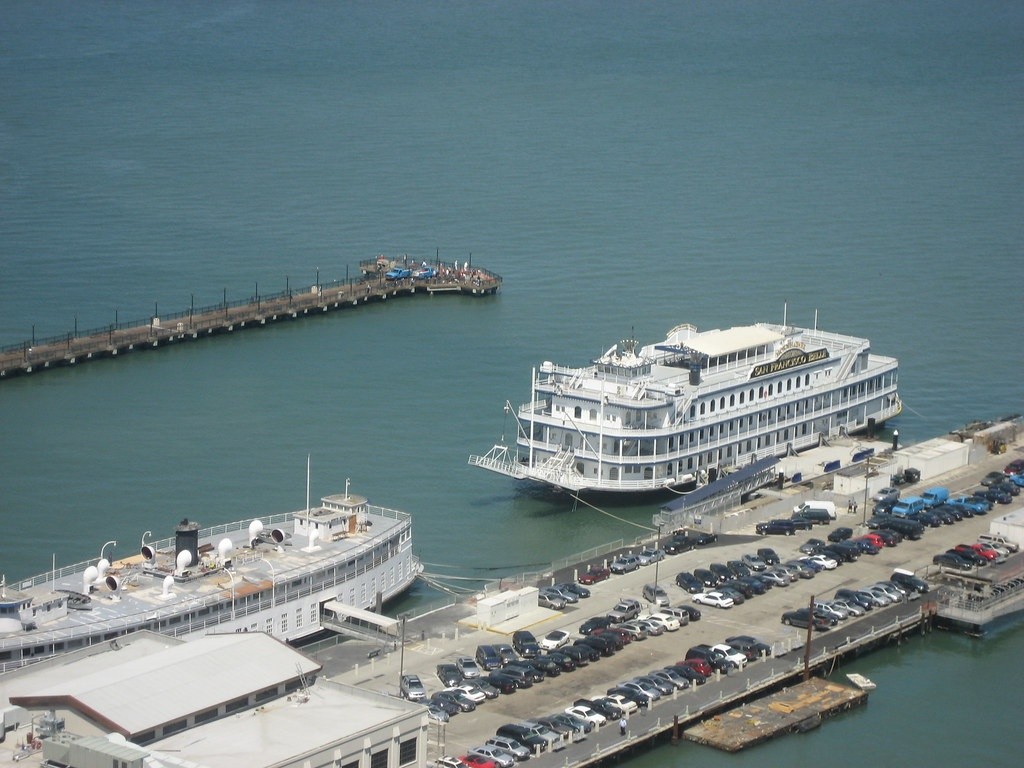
[0,489,425,678]
[474,320,902,506]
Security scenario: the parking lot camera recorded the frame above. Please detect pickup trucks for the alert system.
[412,265,435,279]
[386,266,412,280]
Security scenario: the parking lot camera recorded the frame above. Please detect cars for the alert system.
[635,551,663,565]
[547,585,579,603]
[399,673,422,699]
[698,532,716,546]
[577,568,609,582]
[678,458,1023,634]
[664,535,695,554]
[430,599,773,768]
[537,590,563,609]
[609,556,637,571]
[641,581,670,605]
[559,581,591,595]
